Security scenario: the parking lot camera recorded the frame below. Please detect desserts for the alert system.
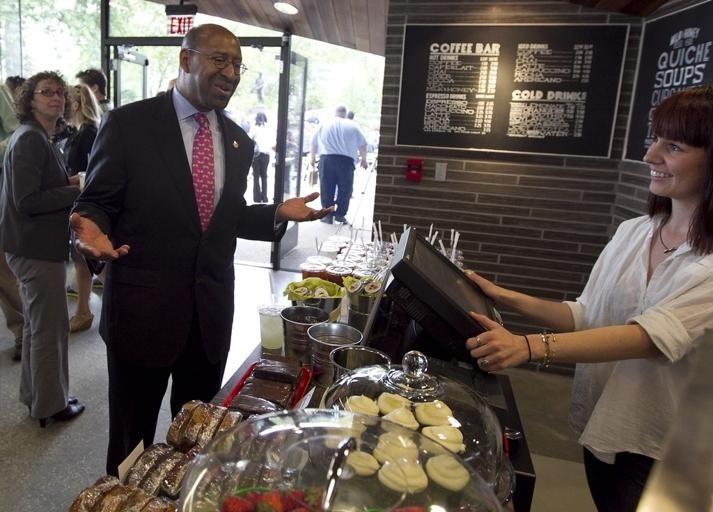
[325,391,469,493]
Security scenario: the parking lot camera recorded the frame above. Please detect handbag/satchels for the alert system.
[309,165,317,185]
[253,135,260,159]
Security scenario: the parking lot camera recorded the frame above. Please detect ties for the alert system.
[191,112,215,230]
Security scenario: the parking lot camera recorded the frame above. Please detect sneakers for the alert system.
[91,277,104,288]
[66,282,78,298]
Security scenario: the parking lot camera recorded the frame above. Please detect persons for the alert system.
[272,117,295,196]
[0,65,113,362]
[68,22,336,482]
[346,111,353,121]
[245,111,272,203]
[2,71,85,430]
[309,106,367,225]
[464,81,712,512]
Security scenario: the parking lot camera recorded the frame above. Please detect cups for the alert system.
[78,171,86,191]
[280,306,390,388]
[257,306,285,350]
[299,233,394,285]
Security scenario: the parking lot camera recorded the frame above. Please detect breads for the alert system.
[68,399,304,512]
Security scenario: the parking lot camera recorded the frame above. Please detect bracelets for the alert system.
[522,335,532,362]
[537,332,556,369]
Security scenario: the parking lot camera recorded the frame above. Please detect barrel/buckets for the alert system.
[279,278,391,386]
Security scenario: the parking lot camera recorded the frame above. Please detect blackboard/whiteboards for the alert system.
[621,0,713,165]
[395,23,631,159]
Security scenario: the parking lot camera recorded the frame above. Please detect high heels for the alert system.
[19,396,79,417]
[69,311,94,332]
[39,404,85,428]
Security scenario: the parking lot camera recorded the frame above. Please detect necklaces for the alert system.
[656,219,679,254]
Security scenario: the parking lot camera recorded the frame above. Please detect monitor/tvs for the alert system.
[385,225,499,339]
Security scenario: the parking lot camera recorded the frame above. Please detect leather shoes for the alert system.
[334,215,349,224]
[11,338,22,361]
[321,216,334,225]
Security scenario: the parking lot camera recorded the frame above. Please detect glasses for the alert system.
[31,88,66,98]
[187,48,248,76]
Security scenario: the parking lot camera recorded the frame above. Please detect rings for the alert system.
[479,359,488,369]
[475,335,480,347]
